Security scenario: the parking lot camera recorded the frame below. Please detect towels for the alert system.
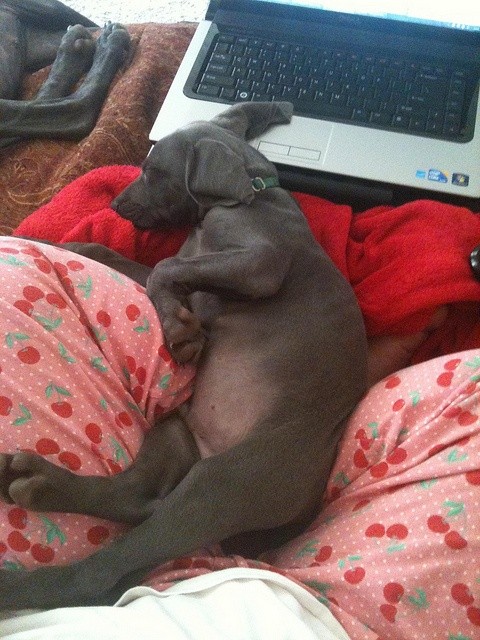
[11,164,480,338]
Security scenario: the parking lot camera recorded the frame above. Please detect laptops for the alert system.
[149,0,480,199]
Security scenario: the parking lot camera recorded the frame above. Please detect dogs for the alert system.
[1,0,128,146]
[0,99,373,613]
